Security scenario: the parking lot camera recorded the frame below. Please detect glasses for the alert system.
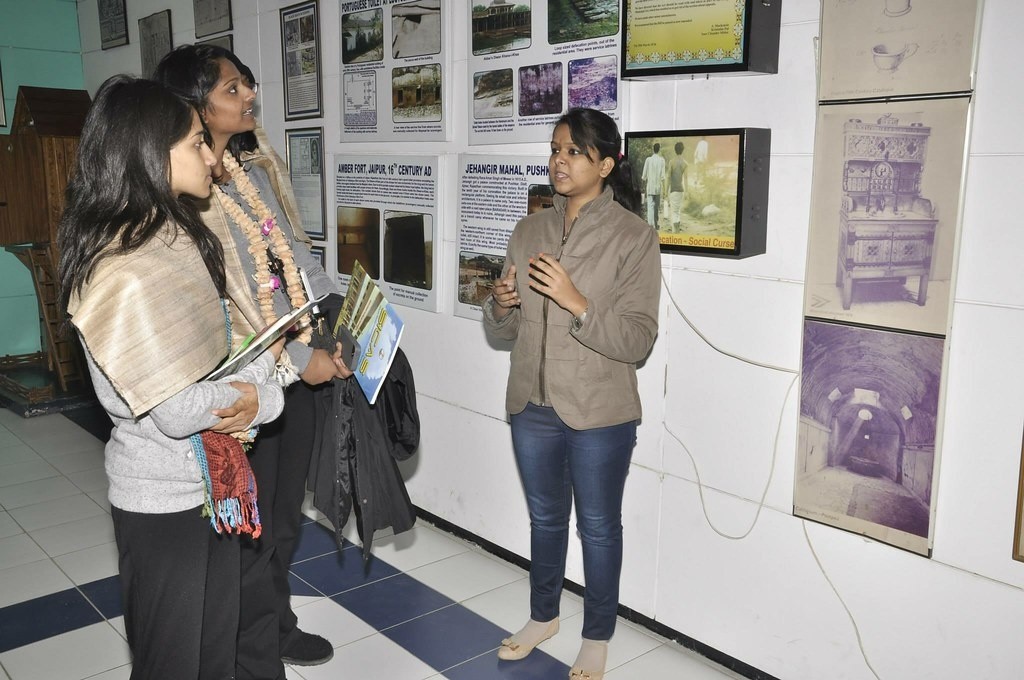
[250,83,260,93]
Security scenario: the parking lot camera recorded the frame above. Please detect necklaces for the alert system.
[211,148,314,349]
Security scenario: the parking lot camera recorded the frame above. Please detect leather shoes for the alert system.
[568,642,607,680]
[498,617,559,660]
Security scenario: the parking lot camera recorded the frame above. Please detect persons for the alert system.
[481,108,662,680]
[641,135,708,234]
[155,41,354,680]
[54,72,287,680]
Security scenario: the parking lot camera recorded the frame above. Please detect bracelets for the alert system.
[578,307,588,324]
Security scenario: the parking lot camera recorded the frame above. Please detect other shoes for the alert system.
[281,626,334,666]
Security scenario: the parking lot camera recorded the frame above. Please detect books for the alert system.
[204,293,330,381]
[331,259,404,405]
